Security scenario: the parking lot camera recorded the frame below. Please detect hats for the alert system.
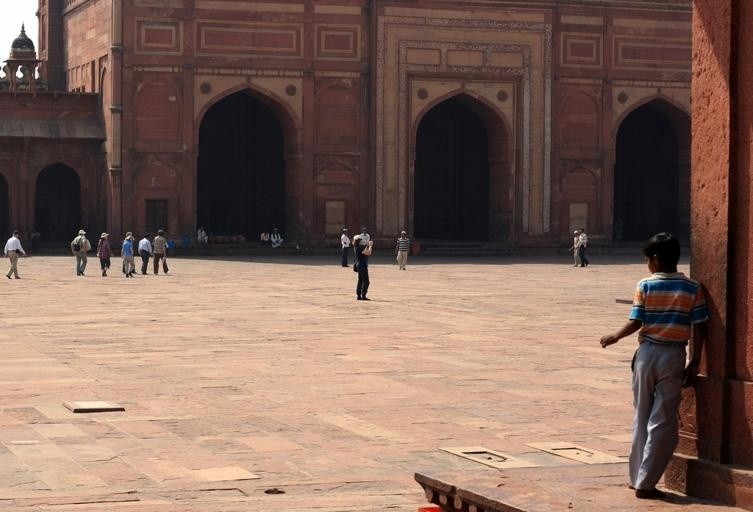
[125,236,134,240]
[78,230,86,235]
[101,233,109,238]
[354,234,363,240]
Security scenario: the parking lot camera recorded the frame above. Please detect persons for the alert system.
[352,234,374,301]
[569,231,581,265]
[1,228,284,279]
[600,230,710,500]
[576,228,592,267]
[339,226,411,269]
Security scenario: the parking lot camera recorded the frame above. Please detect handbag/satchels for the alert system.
[73,243,81,251]
